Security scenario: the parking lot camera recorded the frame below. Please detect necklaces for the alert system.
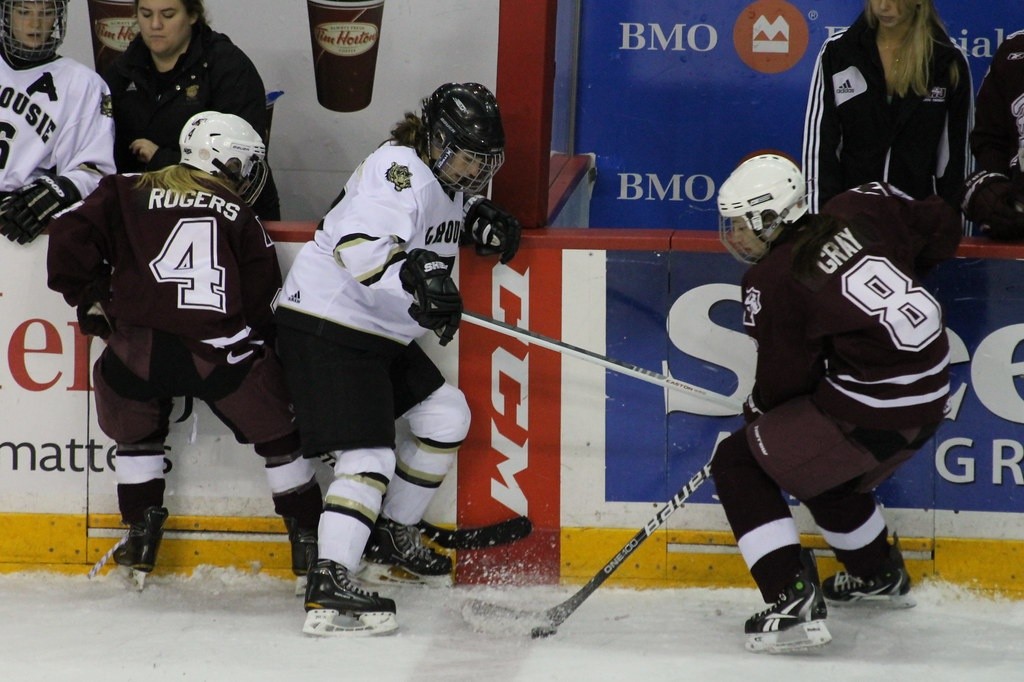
[879,34,912,63]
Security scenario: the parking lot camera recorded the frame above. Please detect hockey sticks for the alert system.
[316,449,534,551]
[460,462,713,634]
[458,310,745,410]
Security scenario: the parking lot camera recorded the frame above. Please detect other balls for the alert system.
[531,627,558,640]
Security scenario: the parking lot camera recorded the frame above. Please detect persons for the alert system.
[707,153,962,654]
[958,29,1024,242]
[270,83,522,638]
[802,0,978,237]
[48,109,323,599]
[104,0,281,221]
[0,0,117,245]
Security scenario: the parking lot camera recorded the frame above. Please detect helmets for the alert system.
[0,0,68,64]
[421,82,506,194]
[717,153,809,264]
[178,110,269,206]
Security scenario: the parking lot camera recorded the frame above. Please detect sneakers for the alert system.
[288,534,323,597]
[744,549,831,653]
[110,505,169,593]
[354,514,454,589]
[302,558,398,638]
[822,532,917,611]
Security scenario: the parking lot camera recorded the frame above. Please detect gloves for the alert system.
[742,395,762,424]
[460,198,521,265]
[398,248,462,347]
[75,299,110,339]
[1,174,81,245]
